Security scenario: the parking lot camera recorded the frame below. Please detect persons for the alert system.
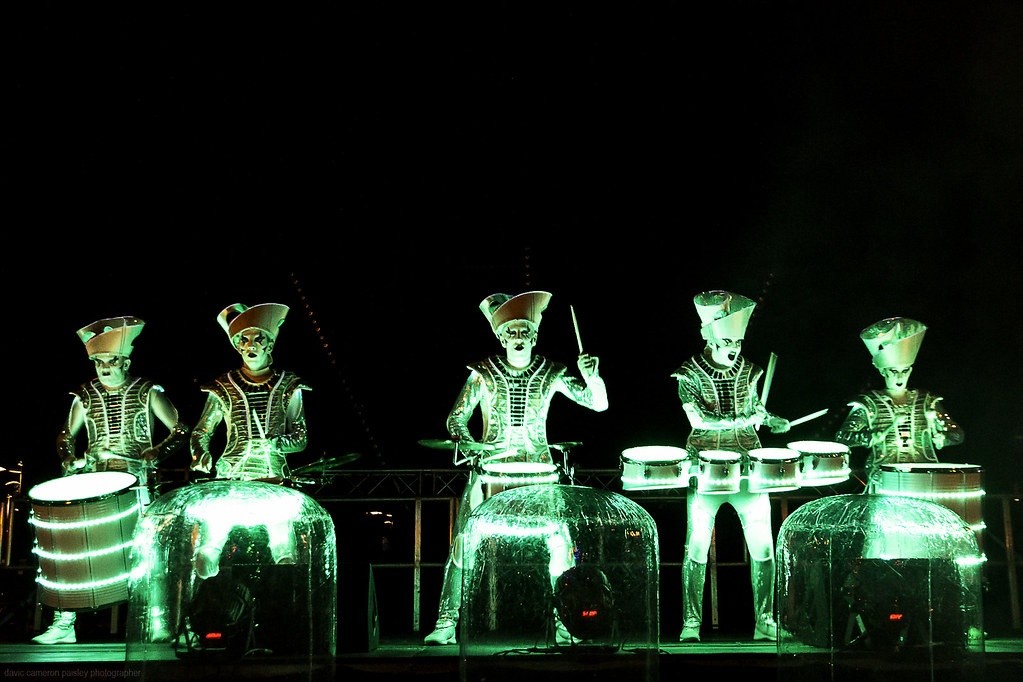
[32,315,188,642]
[426,290,608,648]
[839,315,964,562]
[669,288,796,644]
[191,298,311,483]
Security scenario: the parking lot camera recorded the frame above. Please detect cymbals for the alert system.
[547,441,585,451]
[0,496,30,504]
[290,451,363,474]
[128,478,176,491]
[418,437,496,451]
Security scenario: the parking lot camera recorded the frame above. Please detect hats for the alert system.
[76,316,146,360]
[216,303,290,349]
[478,290,553,338]
[694,289,757,340]
[860,316,928,370]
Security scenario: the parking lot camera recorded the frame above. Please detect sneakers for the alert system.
[753,613,793,641]
[151,625,171,643]
[555,621,583,644]
[679,617,700,642]
[968,627,987,652]
[424,619,457,644]
[32,625,77,644]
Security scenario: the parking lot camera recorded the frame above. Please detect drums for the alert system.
[26,472,144,612]
[194,477,284,486]
[620,446,691,491]
[786,440,852,487]
[747,448,804,493]
[487,461,560,501]
[696,448,741,495]
[880,462,983,563]
